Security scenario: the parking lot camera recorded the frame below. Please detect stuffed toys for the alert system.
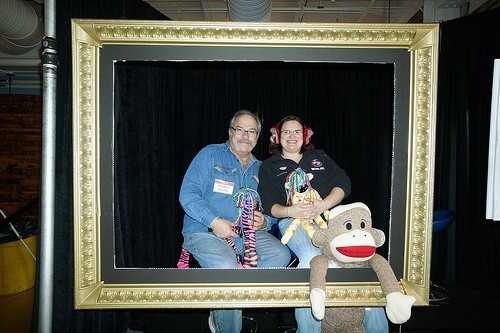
[310,202,416,333]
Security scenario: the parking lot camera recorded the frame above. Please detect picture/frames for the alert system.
[71,18,441,309]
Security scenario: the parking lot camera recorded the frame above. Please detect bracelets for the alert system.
[323,200,328,210]
[287,206,290,219]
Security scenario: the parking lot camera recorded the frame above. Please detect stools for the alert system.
[429,210,453,301]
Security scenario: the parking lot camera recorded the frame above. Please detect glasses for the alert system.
[229,125,259,136]
[280,130,304,135]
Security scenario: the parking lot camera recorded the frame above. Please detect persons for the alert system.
[257,115,390,333]
[178,109,292,333]
